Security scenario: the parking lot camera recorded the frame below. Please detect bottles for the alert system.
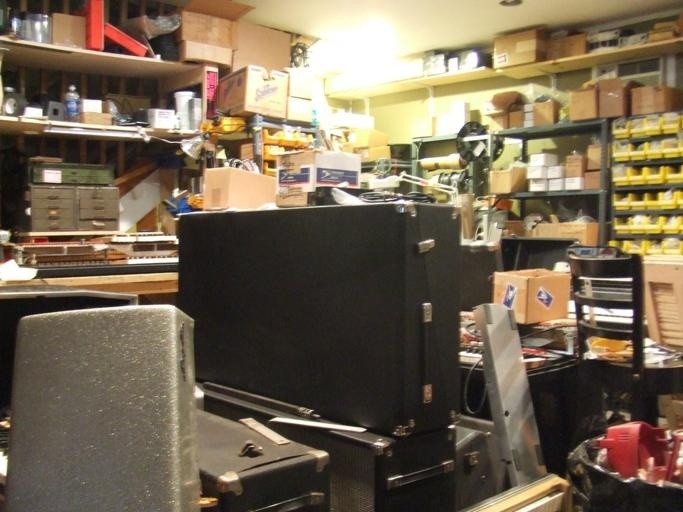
[1,88,18,116]
[64,84,79,120]
[0,231,23,266]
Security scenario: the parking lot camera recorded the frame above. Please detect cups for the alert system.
[173,90,192,131]
[8,9,48,42]
[24,107,42,118]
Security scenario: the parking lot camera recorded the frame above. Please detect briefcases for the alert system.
[178,205,463,398]
[175,396,498,511]
[175,205,498,511]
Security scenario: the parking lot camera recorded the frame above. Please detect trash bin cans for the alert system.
[566,434,682,511]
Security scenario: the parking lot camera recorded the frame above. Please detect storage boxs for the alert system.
[492,266,571,325]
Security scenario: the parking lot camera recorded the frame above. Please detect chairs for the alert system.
[568,252,682,429]
